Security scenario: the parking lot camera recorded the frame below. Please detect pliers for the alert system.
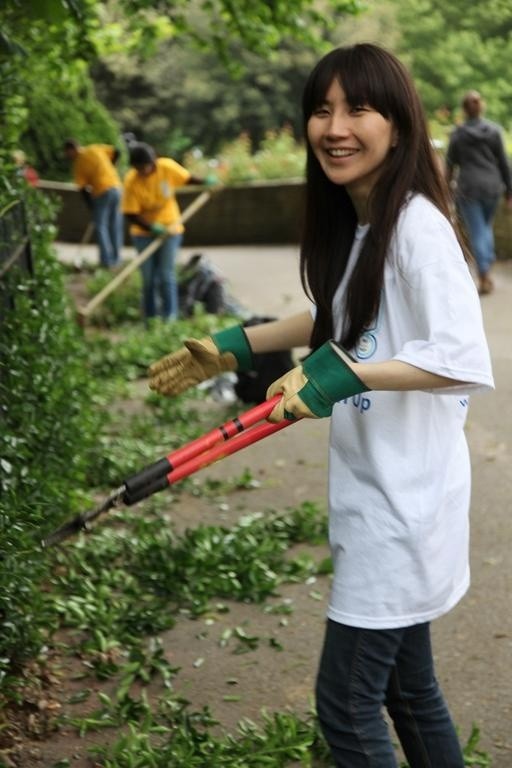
[39,393,304,550]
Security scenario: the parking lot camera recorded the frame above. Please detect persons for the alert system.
[118,141,221,331]
[61,135,128,269]
[441,89,511,297]
[146,41,498,765]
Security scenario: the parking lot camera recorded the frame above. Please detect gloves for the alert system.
[145,324,251,396]
[206,176,221,190]
[149,223,169,237]
[267,339,372,421]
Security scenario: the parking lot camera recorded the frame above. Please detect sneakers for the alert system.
[479,275,493,293]
